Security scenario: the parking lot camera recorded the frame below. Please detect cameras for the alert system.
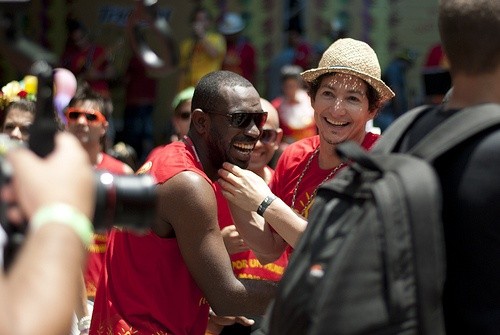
[5,64,175,243]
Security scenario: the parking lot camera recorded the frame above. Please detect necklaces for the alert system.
[293,147,344,213]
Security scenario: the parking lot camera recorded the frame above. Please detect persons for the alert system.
[0,0,500,335]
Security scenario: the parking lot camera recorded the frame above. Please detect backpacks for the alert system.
[252,102,500,335]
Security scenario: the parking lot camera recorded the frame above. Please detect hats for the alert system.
[300,37,395,104]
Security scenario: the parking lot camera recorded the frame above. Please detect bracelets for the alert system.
[29,202,94,248]
[79,315,93,330]
[257,195,277,216]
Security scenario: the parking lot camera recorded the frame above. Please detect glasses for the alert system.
[174,111,191,120]
[65,108,106,123]
[199,109,268,128]
[258,129,279,145]
